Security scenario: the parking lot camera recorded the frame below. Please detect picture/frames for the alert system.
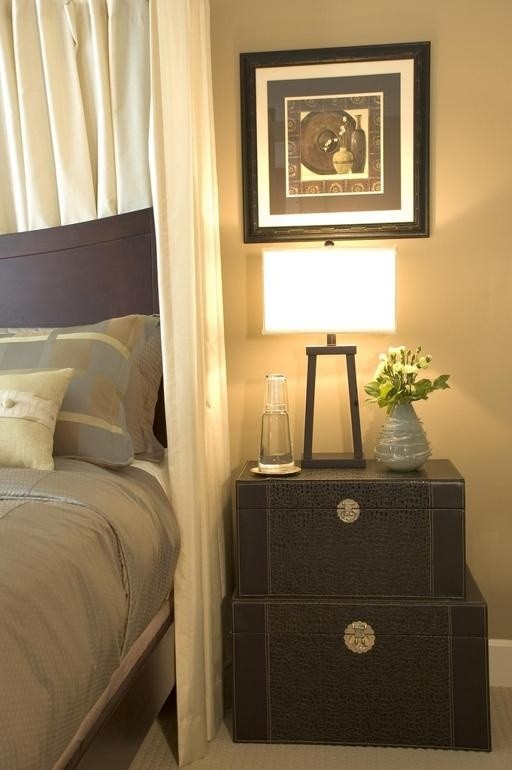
[239,39,432,243]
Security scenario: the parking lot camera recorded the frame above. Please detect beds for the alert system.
[0,208,176,770]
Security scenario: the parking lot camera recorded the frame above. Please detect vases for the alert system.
[373,401,432,472]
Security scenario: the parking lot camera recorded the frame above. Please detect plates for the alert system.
[249,466,301,475]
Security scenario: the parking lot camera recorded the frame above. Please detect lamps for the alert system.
[262,234,398,471]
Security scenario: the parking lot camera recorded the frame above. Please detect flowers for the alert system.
[363,344,451,415]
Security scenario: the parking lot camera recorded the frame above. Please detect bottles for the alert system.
[257,404,294,471]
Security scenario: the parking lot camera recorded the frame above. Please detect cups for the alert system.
[262,372,290,414]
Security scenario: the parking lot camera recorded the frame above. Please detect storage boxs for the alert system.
[230,561,491,751]
[235,458,465,600]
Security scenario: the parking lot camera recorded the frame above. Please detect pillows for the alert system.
[0,314,166,471]
